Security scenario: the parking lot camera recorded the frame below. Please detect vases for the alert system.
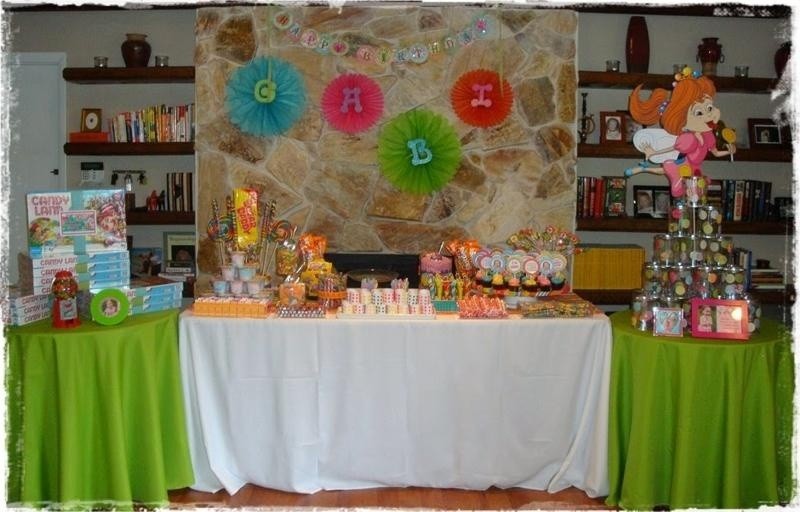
[626,16,649,73]
[696,37,725,76]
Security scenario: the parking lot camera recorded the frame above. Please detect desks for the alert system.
[4,307,182,508]
[178,288,613,501]
[610,309,793,509]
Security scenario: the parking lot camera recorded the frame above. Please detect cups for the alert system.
[674,65,685,73]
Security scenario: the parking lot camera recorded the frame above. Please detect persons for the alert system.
[606,117,622,140]
[697,305,717,332]
[637,191,652,212]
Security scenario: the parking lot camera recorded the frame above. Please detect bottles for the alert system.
[51,271,78,297]
[94,55,107,67]
[630,175,762,336]
[606,59,620,73]
[154,55,168,68]
[734,65,749,79]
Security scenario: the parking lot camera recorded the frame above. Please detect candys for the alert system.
[720,128,737,163]
[194,182,594,317]
[675,164,692,185]
[632,177,762,335]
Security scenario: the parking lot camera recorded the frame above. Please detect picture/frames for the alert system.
[617,110,646,146]
[163,231,195,272]
[599,112,627,148]
[747,118,785,149]
[691,297,750,341]
[632,184,675,219]
[652,307,684,338]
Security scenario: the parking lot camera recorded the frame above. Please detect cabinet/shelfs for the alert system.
[61,64,195,296]
[572,70,792,305]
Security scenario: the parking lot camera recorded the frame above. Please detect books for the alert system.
[109,103,195,143]
[577,176,604,218]
[167,171,191,214]
[157,231,195,282]
[719,236,785,292]
[706,179,772,224]
